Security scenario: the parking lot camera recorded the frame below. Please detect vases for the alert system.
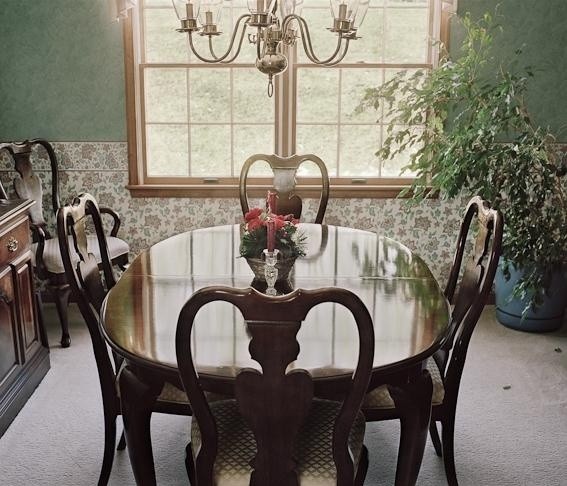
[244,256,297,282]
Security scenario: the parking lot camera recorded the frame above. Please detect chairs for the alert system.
[56,193,234,482]
[0,137,129,347]
[318,196,503,482]
[175,286,375,482]
[239,154,330,225]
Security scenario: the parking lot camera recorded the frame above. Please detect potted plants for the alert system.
[342,0,566,334]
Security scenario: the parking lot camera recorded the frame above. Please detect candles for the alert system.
[266,221,275,255]
[266,190,277,215]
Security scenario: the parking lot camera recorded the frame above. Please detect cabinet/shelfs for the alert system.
[0,198,51,440]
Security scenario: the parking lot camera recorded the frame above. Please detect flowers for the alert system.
[235,207,307,263]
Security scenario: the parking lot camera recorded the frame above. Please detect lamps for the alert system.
[172,1,371,98]
